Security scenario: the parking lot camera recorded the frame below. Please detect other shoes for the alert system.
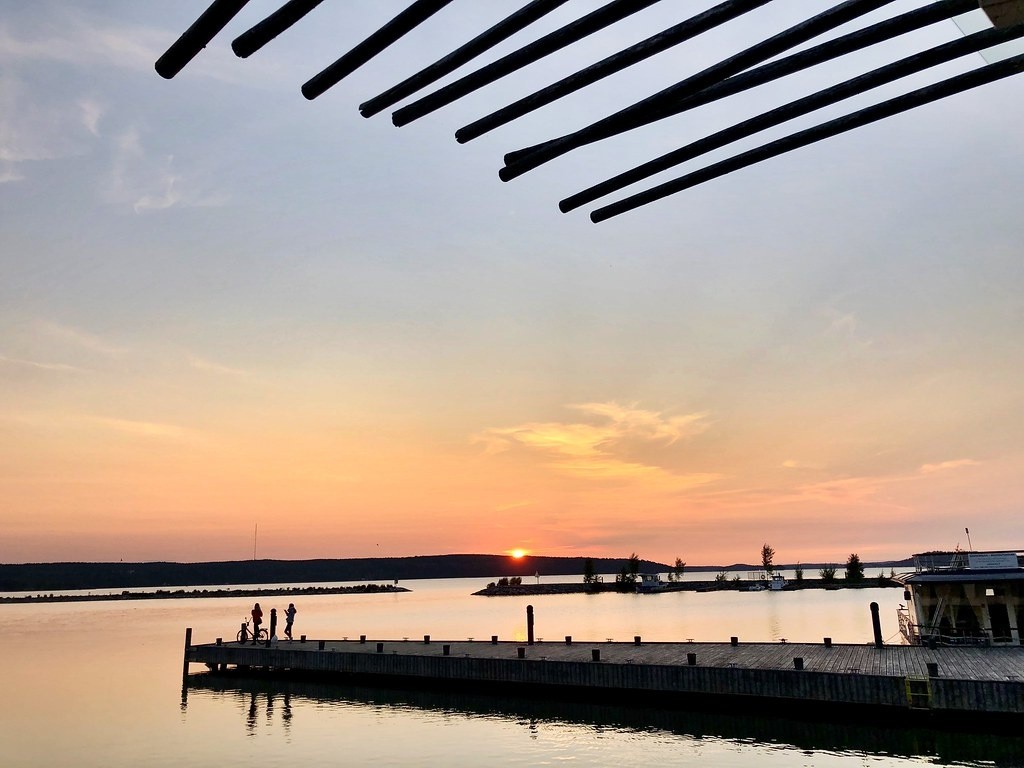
[288,637,293,644]
[251,642,256,645]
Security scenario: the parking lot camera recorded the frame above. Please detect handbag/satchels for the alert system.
[255,614,262,624]
[271,635,278,648]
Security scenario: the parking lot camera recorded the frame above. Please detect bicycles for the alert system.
[237,616,269,644]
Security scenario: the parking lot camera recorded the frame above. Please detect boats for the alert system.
[890,548,1024,647]
[635,574,669,594]
[768,573,788,590]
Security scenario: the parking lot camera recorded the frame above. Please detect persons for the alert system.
[284,603,297,641]
[251,603,263,645]
